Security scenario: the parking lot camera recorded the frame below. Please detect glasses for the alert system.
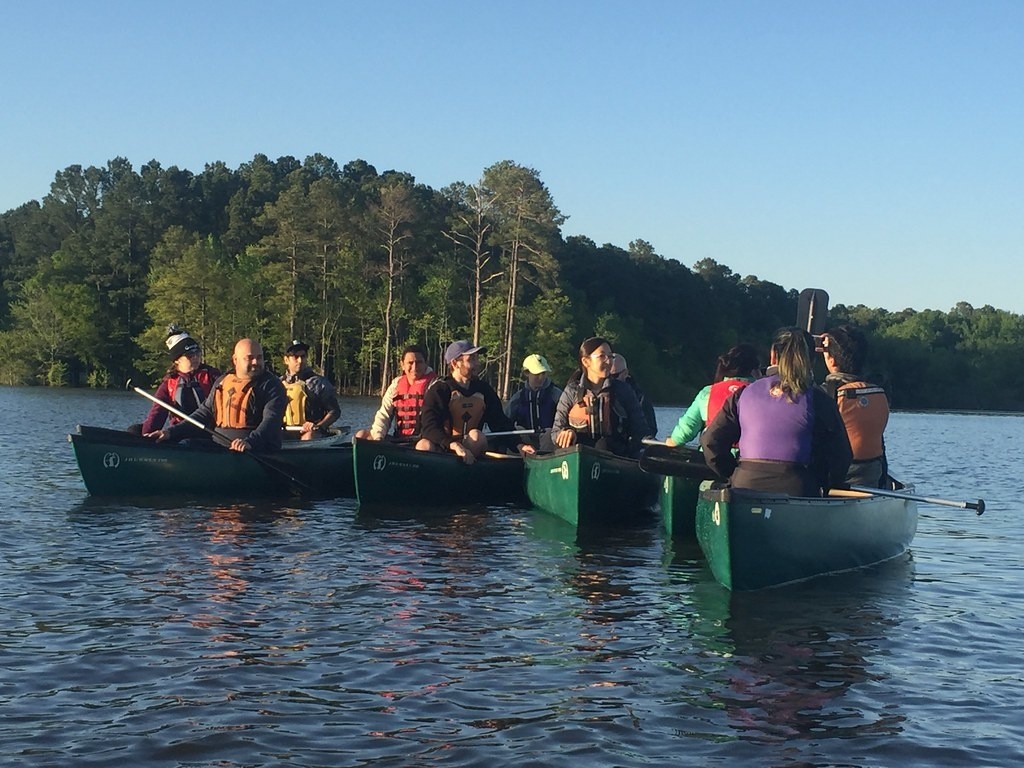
[585,353,614,361]
[180,349,203,360]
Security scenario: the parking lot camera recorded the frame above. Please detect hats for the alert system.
[444,340,488,365]
[609,353,627,374]
[285,340,308,355]
[523,354,552,374]
[166,324,199,363]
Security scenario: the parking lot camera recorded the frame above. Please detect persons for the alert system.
[551,338,646,451]
[815,321,889,493]
[606,353,658,459]
[415,340,537,464]
[664,344,762,459]
[700,327,854,497]
[144,338,289,451]
[275,339,342,440]
[504,354,564,455]
[355,345,439,442]
[128,327,225,439]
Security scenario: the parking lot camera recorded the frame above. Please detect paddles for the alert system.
[639,445,874,498]
[837,482,987,517]
[329,429,535,447]
[282,426,352,436]
[125,379,316,492]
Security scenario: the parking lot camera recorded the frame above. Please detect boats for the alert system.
[352,435,524,508]
[657,475,917,594]
[524,444,661,528]
[67,425,354,497]
